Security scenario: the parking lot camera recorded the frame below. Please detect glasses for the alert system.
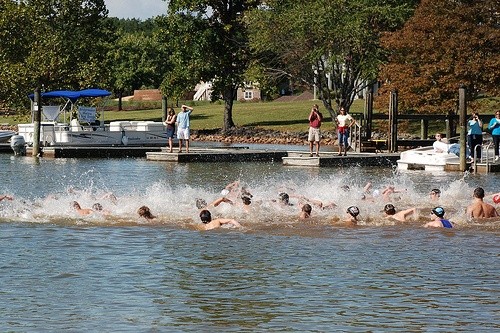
[340,109,345,111]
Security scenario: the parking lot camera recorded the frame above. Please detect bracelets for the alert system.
[5,195,6,197]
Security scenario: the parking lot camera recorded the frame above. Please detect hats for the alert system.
[432,206,444,217]
[493,194,500,204]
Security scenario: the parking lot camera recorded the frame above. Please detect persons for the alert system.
[165,108,177,153]
[176,104,193,153]
[467,112,483,163]
[138,206,155,219]
[335,106,354,156]
[468,187,498,218]
[343,182,417,222]
[308,103,322,157]
[193,181,254,215]
[430,189,441,200]
[488,110,500,161]
[197,210,242,230]
[494,195,500,205]
[70,201,94,214]
[0,195,14,202]
[433,132,473,162]
[423,207,454,229]
[93,203,105,212]
[278,193,337,219]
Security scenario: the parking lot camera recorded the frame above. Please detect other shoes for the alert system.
[168,151,172,153]
[316,155,319,157]
[309,154,314,157]
[178,151,182,153]
[344,154,347,156]
[186,151,189,153]
[337,154,342,156]
